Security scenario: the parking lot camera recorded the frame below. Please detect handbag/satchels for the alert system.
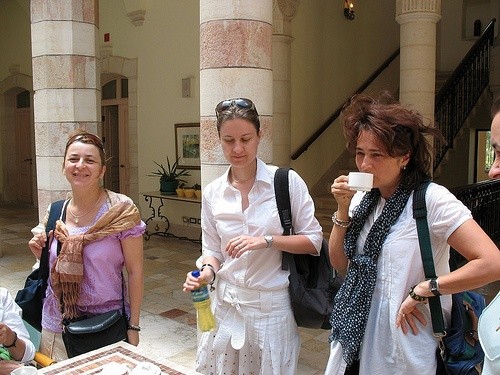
[412,180,488,375]
[274,166,343,331]
[13,199,66,332]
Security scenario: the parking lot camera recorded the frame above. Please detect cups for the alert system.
[345,172,374,192]
[10,365,39,375]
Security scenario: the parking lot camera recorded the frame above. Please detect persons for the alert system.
[325,90,500,375]
[0,287,36,375]
[28,130,147,359]
[183,97,323,375]
[478,95,500,375]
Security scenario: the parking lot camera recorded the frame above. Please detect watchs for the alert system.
[429,277,443,296]
[264,234,273,248]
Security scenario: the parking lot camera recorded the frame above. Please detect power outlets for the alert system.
[183,216,201,225]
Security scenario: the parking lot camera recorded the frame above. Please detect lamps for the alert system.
[343,1,355,21]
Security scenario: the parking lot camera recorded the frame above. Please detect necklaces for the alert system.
[69,189,102,224]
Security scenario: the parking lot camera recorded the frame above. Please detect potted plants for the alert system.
[145,156,192,194]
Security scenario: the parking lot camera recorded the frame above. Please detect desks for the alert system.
[140,191,201,246]
[38,340,204,375]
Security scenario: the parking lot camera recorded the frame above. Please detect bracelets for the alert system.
[127,323,140,331]
[332,210,352,228]
[201,263,216,292]
[2,331,17,349]
[408,284,427,302]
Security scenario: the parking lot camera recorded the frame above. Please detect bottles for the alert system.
[191,269,216,332]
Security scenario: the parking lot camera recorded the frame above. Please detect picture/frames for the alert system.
[175,123,201,169]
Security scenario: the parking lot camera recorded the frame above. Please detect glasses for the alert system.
[215,98,259,121]
